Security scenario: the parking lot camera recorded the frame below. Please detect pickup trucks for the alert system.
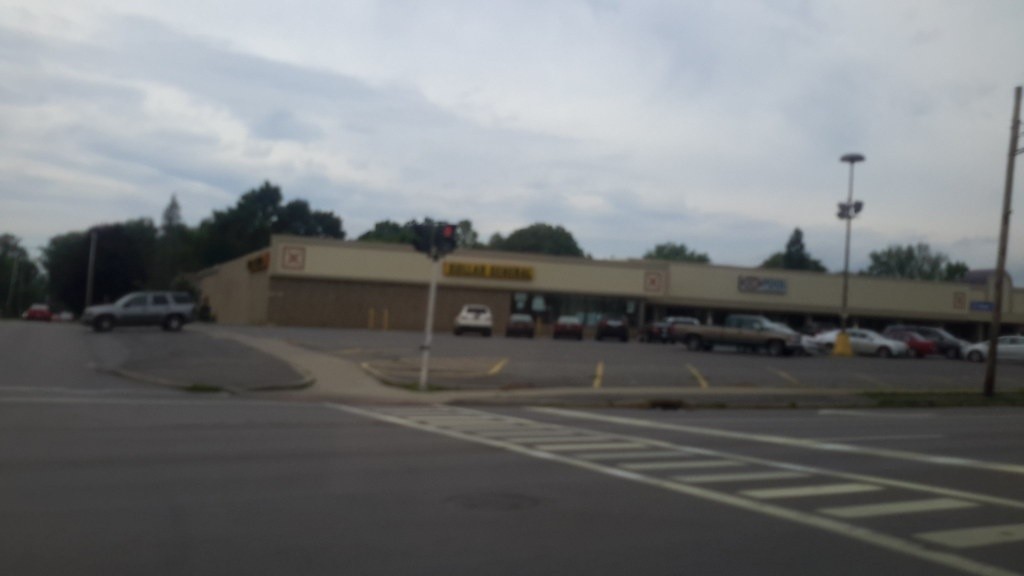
[83,291,195,332]
[671,313,801,355]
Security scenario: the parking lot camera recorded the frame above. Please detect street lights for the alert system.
[839,154,865,334]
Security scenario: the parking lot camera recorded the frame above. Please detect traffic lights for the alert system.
[412,222,432,255]
[436,223,456,256]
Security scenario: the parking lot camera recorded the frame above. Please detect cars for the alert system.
[964,334,1024,363]
[890,324,967,357]
[596,313,628,342]
[886,329,934,358]
[507,313,535,336]
[25,304,51,321]
[815,327,909,359]
[554,316,584,338]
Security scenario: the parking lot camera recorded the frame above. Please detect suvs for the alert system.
[656,316,698,339]
[454,304,493,337]
